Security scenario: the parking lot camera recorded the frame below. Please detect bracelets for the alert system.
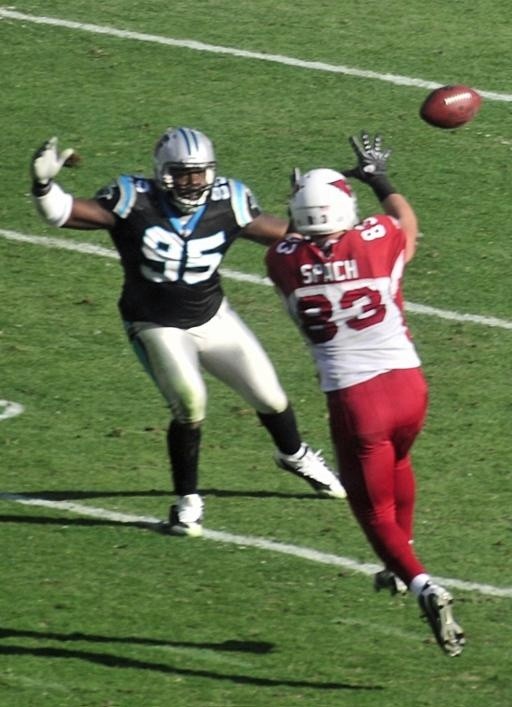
[369,175,401,202]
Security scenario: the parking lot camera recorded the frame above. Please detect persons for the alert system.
[259,125,468,658]
[32,127,350,539]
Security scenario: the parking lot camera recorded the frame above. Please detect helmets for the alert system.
[154,127,217,215]
[287,168,361,239]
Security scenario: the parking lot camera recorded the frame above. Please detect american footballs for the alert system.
[419,83,483,130]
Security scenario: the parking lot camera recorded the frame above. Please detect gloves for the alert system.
[31,135,75,196]
[341,131,399,201]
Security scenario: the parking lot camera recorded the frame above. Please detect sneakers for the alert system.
[375,566,408,597]
[417,580,466,658]
[169,493,205,537]
[271,440,348,500]
